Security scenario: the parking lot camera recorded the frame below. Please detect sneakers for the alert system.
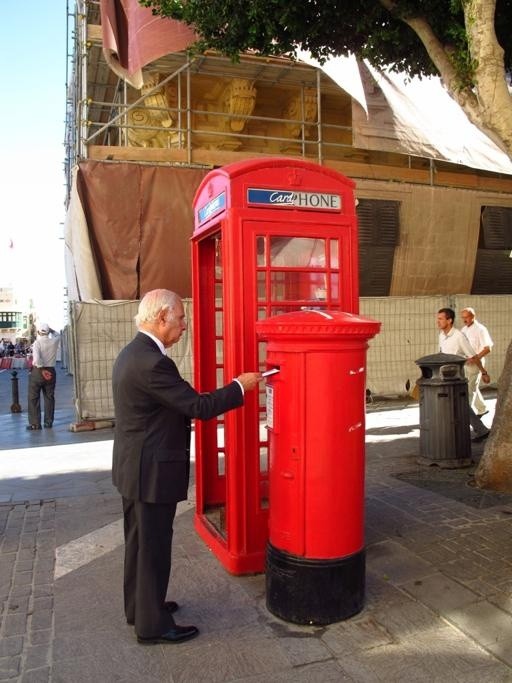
[27,424,42,430]
[43,422,52,428]
[476,410,490,419]
[470,430,491,443]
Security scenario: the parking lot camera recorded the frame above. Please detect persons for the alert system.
[459,305,495,418]
[25,323,61,430]
[107,285,268,644]
[0,336,33,374]
[435,307,492,443]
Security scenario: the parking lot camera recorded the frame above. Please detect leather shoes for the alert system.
[137,625,200,644]
[126,601,178,625]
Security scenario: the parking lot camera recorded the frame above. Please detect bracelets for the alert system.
[482,370,488,375]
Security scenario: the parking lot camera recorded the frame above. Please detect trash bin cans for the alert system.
[415,353,476,470]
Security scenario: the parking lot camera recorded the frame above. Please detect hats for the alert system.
[39,323,50,334]
